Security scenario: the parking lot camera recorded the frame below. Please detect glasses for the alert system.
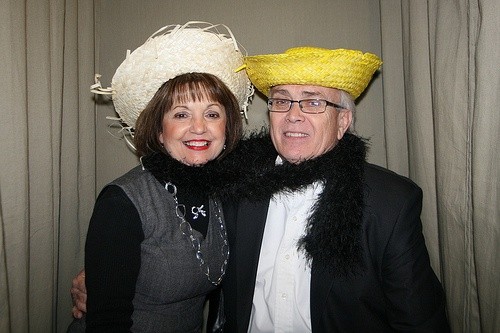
[267,99,346,114]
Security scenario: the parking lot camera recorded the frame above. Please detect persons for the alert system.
[65,20,256,333]
[69,46,447,333]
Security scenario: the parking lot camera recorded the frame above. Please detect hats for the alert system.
[245,45,384,97]
[90,20,254,150]
